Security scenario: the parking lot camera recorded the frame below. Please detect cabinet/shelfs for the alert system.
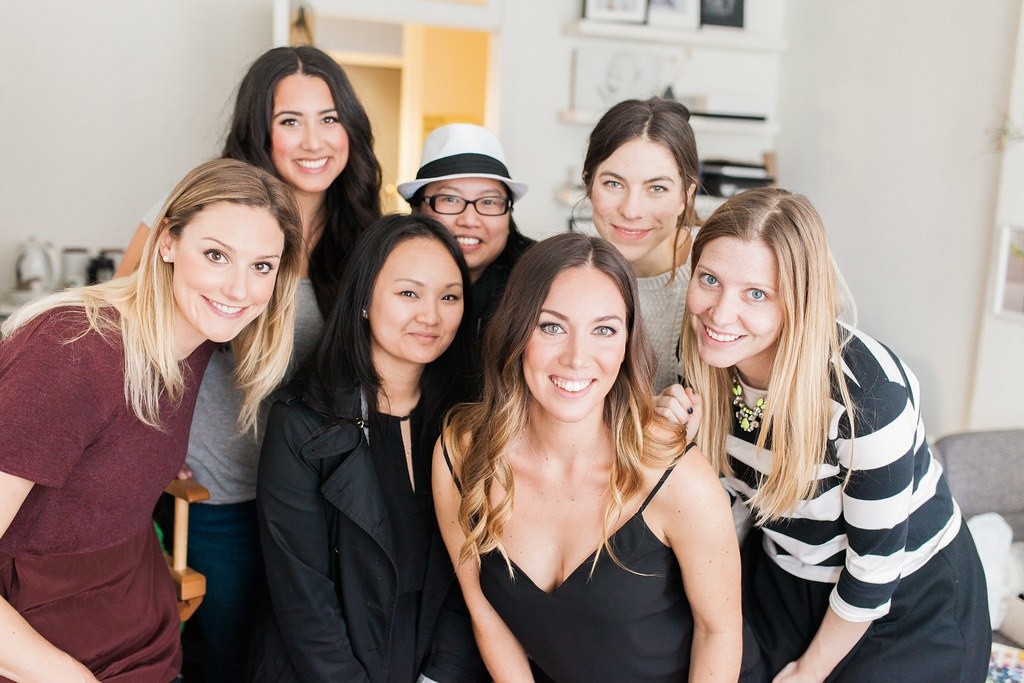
[552,22,786,210]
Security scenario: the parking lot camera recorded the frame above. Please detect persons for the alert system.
[0,45,991,683]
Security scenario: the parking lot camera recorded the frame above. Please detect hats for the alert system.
[397,123,529,202]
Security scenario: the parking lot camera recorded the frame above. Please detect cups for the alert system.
[88,257,114,285]
[62,249,89,288]
[102,250,122,272]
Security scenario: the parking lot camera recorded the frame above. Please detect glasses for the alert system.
[419,194,513,217]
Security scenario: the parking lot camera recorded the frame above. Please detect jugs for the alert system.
[16,237,51,288]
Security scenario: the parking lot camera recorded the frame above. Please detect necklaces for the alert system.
[732,367,769,432]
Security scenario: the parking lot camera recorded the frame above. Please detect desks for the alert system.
[0,301,24,316]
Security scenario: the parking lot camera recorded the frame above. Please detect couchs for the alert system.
[928,430,1024,683]
